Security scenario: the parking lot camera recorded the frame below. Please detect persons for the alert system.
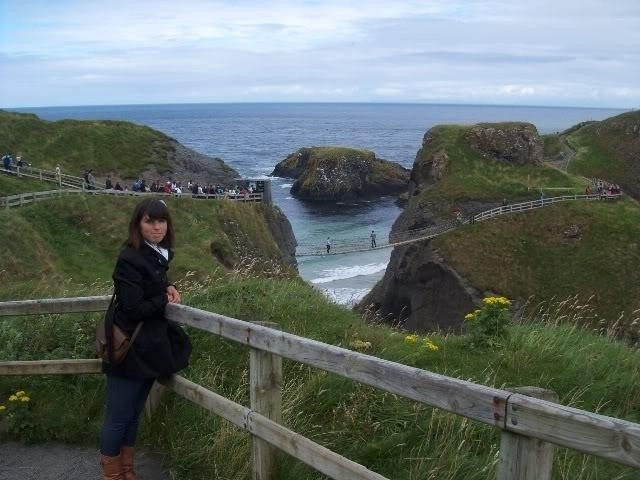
[457,211,462,224]
[1,152,254,199]
[370,230,376,248]
[100,198,181,480]
[326,237,331,253]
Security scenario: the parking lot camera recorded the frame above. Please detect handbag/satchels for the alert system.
[95,320,130,366]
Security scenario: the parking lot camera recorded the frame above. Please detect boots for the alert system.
[99,447,142,480]
